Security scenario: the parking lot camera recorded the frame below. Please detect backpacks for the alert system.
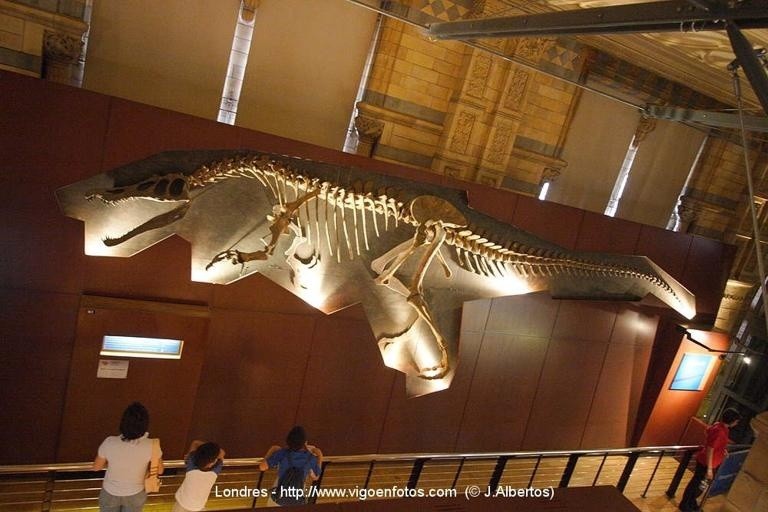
[271,451,312,505]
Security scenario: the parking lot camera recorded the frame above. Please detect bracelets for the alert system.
[707,465,713,473]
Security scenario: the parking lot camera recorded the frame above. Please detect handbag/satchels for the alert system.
[145,471,161,494]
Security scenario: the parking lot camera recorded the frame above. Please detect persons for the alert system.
[258,424,323,506]
[171,438,226,511]
[678,407,741,511]
[93,399,165,511]
